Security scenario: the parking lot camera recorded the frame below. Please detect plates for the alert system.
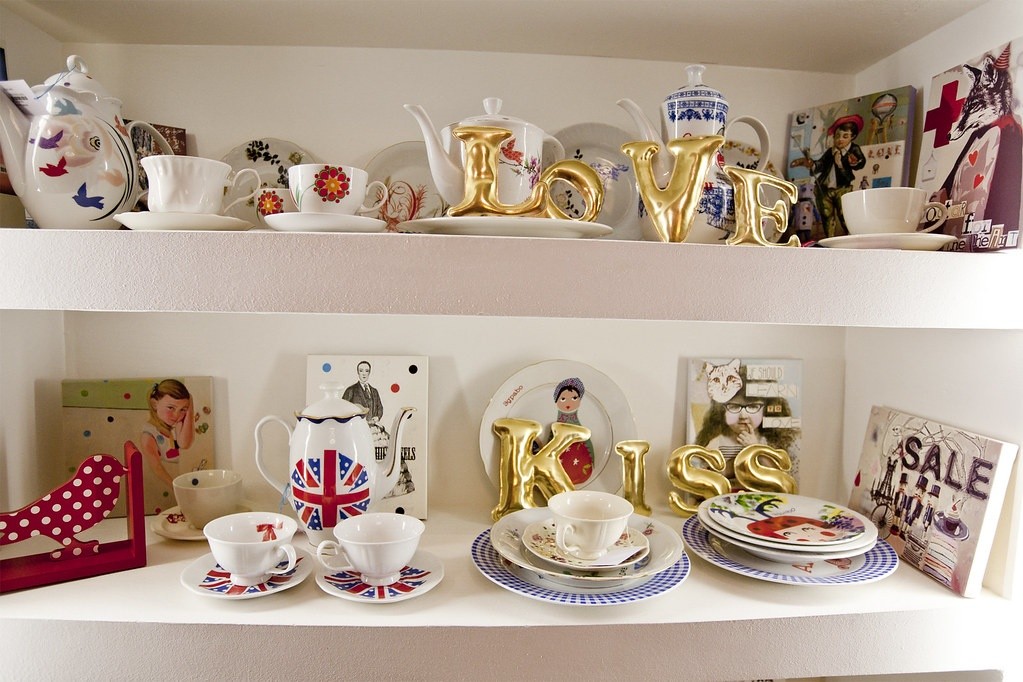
[217,137,316,229]
[817,232,959,251]
[149,492,900,608]
[682,139,789,245]
[478,358,639,509]
[396,217,614,239]
[112,211,255,230]
[361,140,450,234]
[263,212,388,233]
[542,122,644,241]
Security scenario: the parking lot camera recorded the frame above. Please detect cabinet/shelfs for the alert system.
[0,0,1023,682]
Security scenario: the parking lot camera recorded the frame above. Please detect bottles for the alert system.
[24,54,108,229]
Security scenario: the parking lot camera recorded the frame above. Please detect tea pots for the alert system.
[402,96,565,206]
[0,84,176,229]
[253,381,417,554]
[616,65,771,245]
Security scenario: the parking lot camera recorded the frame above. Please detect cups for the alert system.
[253,188,297,221]
[548,490,634,560]
[840,187,948,234]
[287,164,388,215]
[140,154,261,216]
[172,468,243,530]
[202,512,298,586]
[317,513,426,586]
